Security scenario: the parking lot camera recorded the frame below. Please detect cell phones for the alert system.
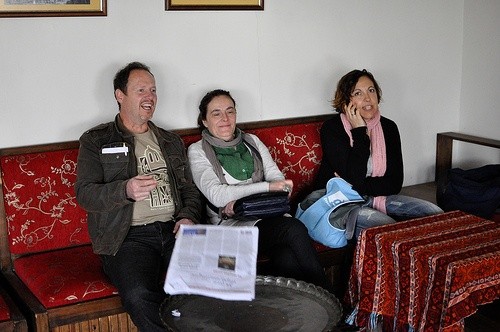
[345,100,357,114]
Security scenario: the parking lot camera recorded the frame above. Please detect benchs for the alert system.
[0,114,354,331]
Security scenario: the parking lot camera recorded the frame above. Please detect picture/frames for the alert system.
[165,0,264,11]
[0,0,107,18]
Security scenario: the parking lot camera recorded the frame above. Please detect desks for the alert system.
[344,210,500,332]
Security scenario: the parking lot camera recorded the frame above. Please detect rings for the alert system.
[230,209,233,210]
[285,185,290,191]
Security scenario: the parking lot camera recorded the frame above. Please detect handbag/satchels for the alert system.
[436,165,500,220]
[233,190,291,221]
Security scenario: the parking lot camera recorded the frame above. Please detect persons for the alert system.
[75,62,205,332]
[189,89,331,294]
[311,70,443,245]
